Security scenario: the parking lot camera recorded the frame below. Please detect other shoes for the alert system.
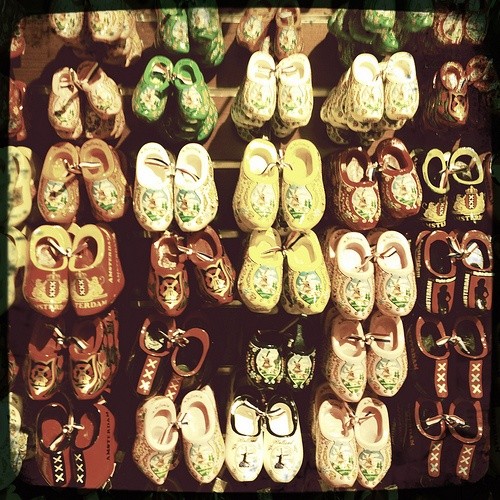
[220,367,306,485]
[421,54,498,138]
[47,62,124,141]
[35,402,118,492]
[47,10,143,68]
[237,227,329,319]
[319,52,419,147]
[403,389,488,488]
[403,313,488,400]
[141,229,233,320]
[324,224,418,322]
[132,140,217,234]
[122,316,213,405]
[11,17,27,60]
[231,138,327,232]
[158,7,191,55]
[309,383,399,490]
[23,225,122,319]
[273,7,306,61]
[35,138,130,224]
[325,136,493,231]
[325,0,500,66]
[132,55,216,141]
[19,309,122,404]
[231,52,313,144]
[235,5,277,53]
[8,77,26,139]
[187,6,225,66]
[6,148,37,227]
[323,310,409,402]
[242,316,312,395]
[130,391,224,486]
[411,229,495,317]
